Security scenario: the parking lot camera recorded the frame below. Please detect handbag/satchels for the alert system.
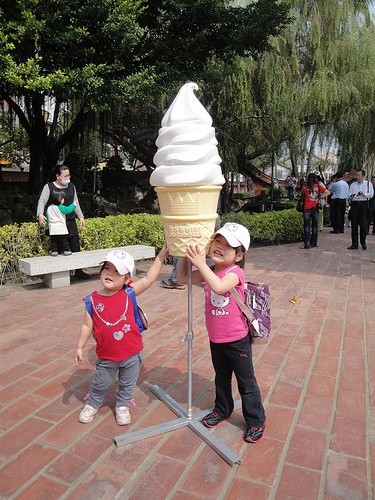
[317,183,323,210]
[296,183,307,212]
[367,207,372,219]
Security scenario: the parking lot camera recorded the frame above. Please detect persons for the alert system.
[44,189,77,256]
[185,222,267,443]
[285,169,375,234]
[347,170,374,250]
[299,173,330,249]
[37,165,91,280]
[159,257,214,290]
[75,241,169,426]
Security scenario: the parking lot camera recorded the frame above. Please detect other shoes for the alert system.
[348,246,358,249]
[330,231,339,233]
[299,245,304,248]
[361,243,367,250]
[51,251,58,256]
[316,242,320,247]
[64,250,72,256]
[75,271,90,279]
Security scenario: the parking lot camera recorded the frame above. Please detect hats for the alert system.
[211,222,251,253]
[99,250,134,277]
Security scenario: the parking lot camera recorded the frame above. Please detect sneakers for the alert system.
[160,278,186,289]
[202,411,224,428]
[115,406,131,425]
[244,422,268,443]
[78,404,99,422]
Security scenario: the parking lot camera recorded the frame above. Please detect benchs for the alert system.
[19,245,155,289]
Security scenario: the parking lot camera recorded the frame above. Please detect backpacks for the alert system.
[224,265,271,339]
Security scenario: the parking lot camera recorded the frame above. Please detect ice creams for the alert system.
[149,82,225,258]
